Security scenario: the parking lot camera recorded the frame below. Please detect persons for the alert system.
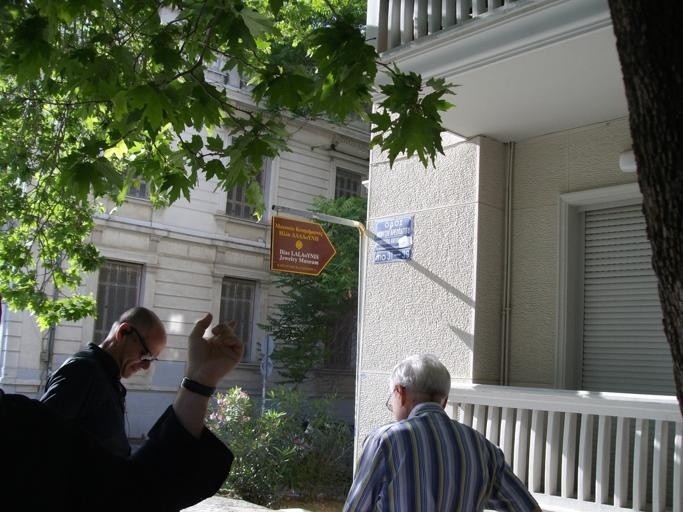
[35,305,167,454]
[0,313,245,511]
[341,354,542,511]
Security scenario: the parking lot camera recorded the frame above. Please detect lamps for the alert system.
[618,145,637,174]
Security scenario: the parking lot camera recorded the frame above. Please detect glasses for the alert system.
[127,323,158,361]
[384,388,397,412]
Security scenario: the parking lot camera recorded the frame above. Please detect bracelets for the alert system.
[180,376,217,398]
